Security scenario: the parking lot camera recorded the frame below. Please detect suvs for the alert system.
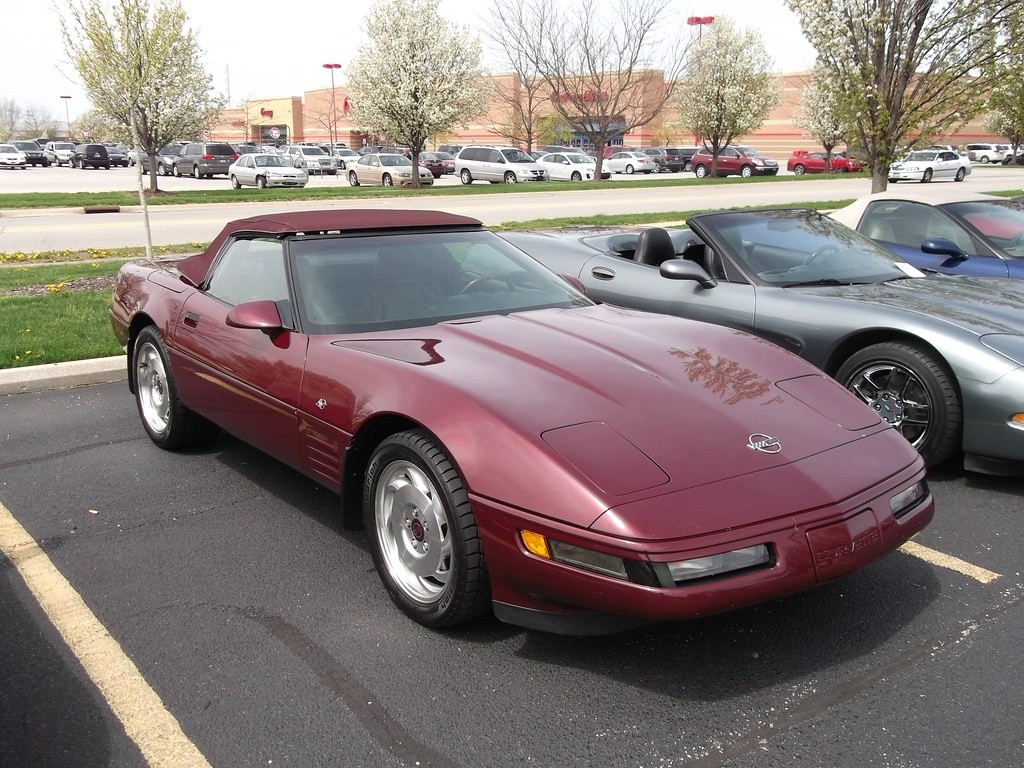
[893,145,1024,166]
[542,145,702,173]
[691,145,779,178]
[438,144,550,185]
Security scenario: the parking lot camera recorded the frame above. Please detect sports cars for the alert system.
[109,209,935,632]
[463,213,1024,477]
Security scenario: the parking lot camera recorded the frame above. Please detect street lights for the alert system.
[687,17,714,146]
[323,64,342,143]
[60,96,71,142]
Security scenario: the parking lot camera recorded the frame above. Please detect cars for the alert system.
[234,139,446,178]
[787,149,868,176]
[0,138,111,170]
[103,140,239,179]
[529,151,612,182]
[602,152,656,174]
[345,153,434,187]
[738,185,1024,279]
[229,153,308,189]
[888,150,972,183]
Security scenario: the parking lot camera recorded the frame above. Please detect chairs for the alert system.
[633,228,676,268]
[705,241,749,282]
